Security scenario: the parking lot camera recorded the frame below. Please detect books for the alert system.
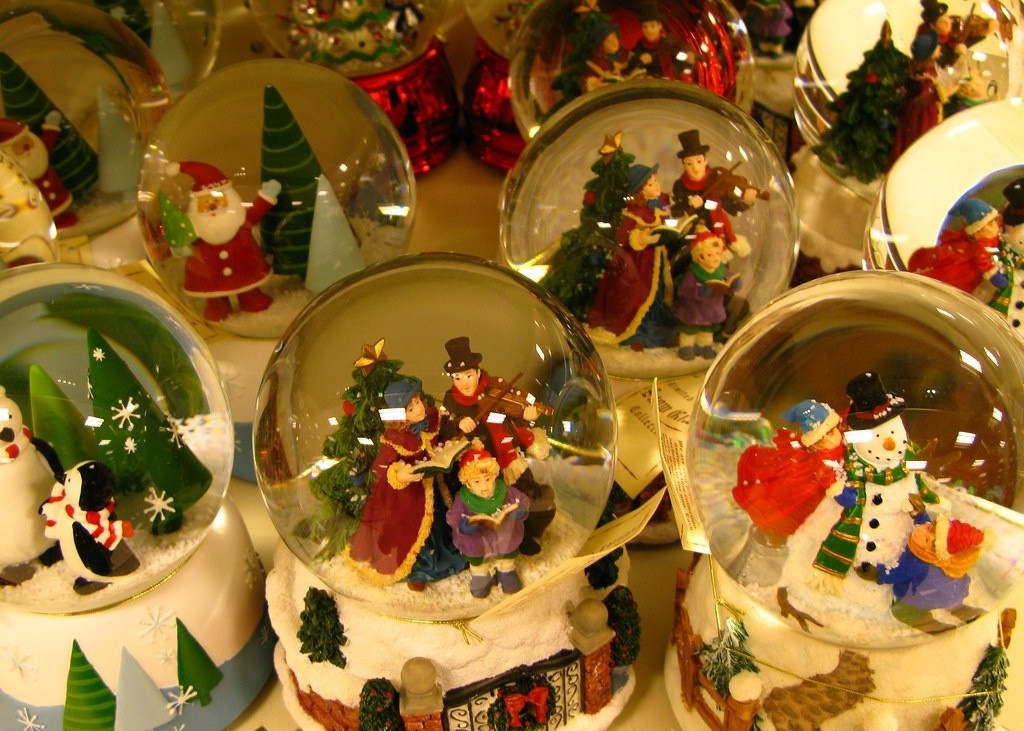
[605,69,646,82]
[648,214,699,246]
[953,54,972,83]
[701,273,740,295]
[411,441,472,472]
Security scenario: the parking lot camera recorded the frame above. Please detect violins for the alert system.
[705,166,770,202]
[950,13,1006,39]
[477,381,554,417]
[661,36,692,55]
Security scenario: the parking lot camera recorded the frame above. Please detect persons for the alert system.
[677,231,740,358]
[948,199,1010,288]
[166,160,282,328]
[860,496,995,630]
[911,0,996,120]
[630,17,700,87]
[673,130,769,346]
[347,378,472,591]
[897,34,972,159]
[440,336,556,554]
[580,22,646,90]
[783,403,841,450]
[448,449,529,594]
[589,162,693,351]
